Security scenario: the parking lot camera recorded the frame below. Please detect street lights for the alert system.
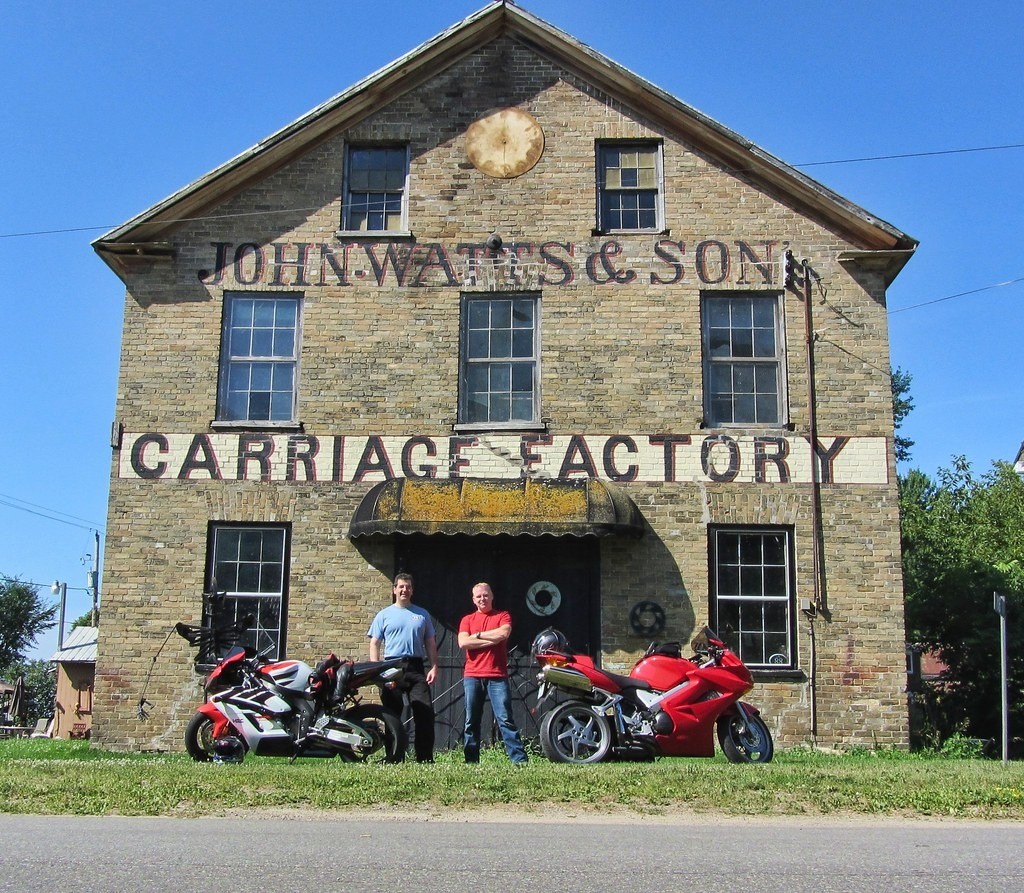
[50,581,67,651]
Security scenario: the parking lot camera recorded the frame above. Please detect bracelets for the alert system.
[476,631,480,639]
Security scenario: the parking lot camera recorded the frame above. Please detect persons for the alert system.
[368,574,440,765]
[457,582,530,764]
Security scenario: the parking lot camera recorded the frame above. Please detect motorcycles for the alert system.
[183,643,411,765]
[532,625,773,763]
